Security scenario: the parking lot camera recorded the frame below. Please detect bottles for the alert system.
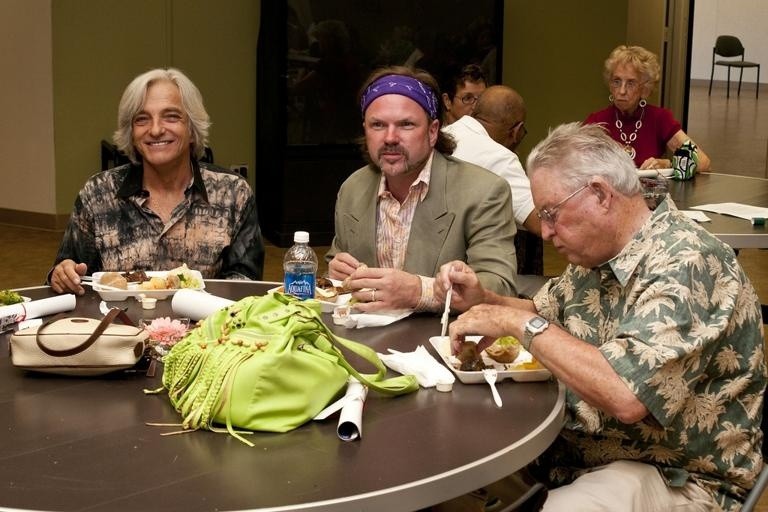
[284,231,318,303]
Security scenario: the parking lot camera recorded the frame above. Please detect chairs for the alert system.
[500,305,768,512]
[708,35,760,100]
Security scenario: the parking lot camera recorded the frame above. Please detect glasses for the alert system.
[609,79,650,89]
[453,94,478,105]
[535,184,590,228]
[508,120,528,140]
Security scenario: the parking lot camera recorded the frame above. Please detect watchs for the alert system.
[521,316,552,354]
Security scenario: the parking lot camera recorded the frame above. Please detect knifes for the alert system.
[441,268,460,336]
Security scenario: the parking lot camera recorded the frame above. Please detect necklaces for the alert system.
[613,108,644,160]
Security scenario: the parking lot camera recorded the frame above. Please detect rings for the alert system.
[372,288,376,302]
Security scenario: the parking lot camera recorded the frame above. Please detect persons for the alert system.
[440,64,487,129]
[436,124,768,512]
[45,67,267,297]
[441,83,570,298]
[579,44,711,172]
[322,64,519,315]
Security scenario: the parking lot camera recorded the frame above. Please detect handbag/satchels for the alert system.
[161,295,420,434]
[673,138,697,179]
[8,309,152,376]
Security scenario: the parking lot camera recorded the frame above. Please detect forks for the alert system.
[483,369,504,408]
